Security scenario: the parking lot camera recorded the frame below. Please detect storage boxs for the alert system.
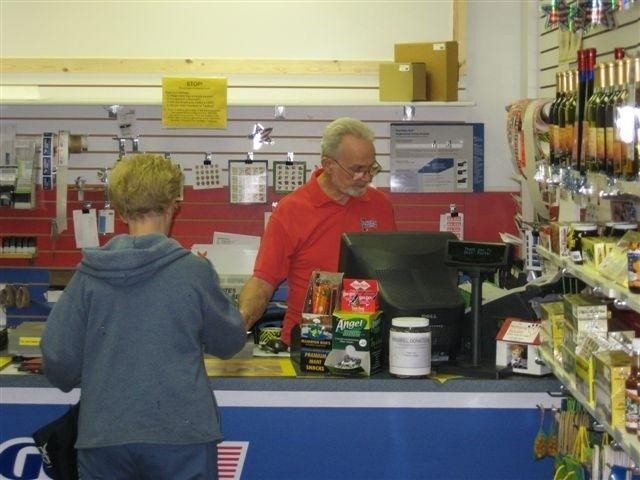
[379,41,459,102]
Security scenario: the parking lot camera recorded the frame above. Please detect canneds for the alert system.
[627,252,640,294]
[568,221,638,265]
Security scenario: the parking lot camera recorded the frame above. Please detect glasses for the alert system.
[321,155,382,181]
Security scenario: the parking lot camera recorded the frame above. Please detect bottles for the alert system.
[549,48,640,180]
[625,354,640,435]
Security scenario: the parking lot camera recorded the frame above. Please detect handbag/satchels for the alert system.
[33,401,79,480]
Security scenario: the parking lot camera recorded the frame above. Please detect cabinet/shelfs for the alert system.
[524,98,638,480]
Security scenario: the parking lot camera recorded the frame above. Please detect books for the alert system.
[18,359,44,372]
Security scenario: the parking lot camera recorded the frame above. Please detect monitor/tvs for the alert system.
[339,231,463,366]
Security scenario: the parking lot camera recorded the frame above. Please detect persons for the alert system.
[39,151,249,480]
[237,117,402,346]
[506,344,526,369]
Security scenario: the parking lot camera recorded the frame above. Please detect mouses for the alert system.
[266,339,287,353]
